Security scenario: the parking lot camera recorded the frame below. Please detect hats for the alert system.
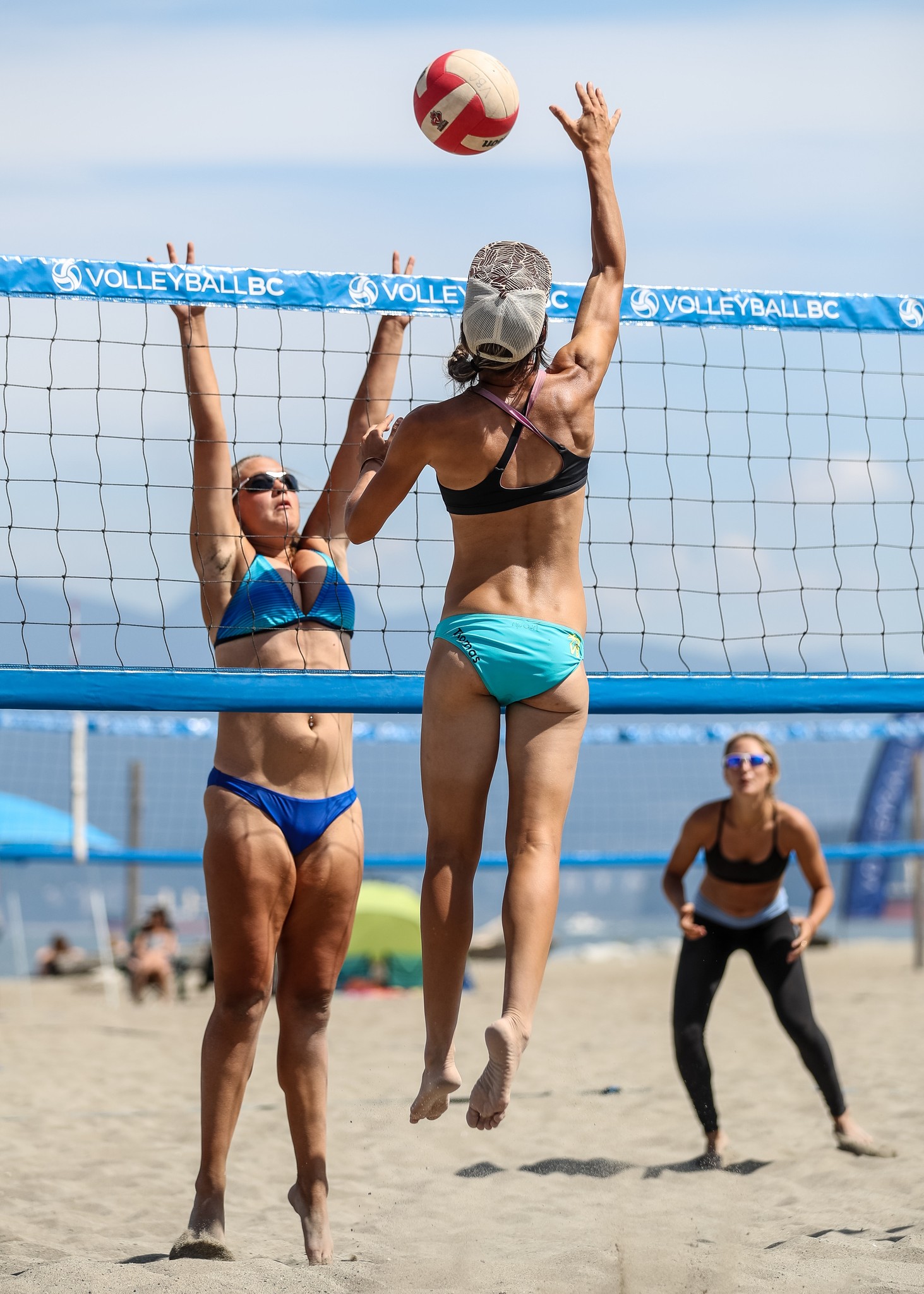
[461,240,554,362]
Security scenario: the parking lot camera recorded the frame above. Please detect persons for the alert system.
[144,242,419,1268]
[661,733,899,1167]
[342,78,628,1129]
[36,900,216,997]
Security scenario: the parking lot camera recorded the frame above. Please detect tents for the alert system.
[0,784,126,1002]
[338,880,476,991]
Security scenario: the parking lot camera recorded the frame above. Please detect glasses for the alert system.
[232,471,299,499]
[724,756,772,768]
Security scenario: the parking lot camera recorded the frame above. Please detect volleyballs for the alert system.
[414,49,518,155]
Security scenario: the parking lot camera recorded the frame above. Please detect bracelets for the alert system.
[359,456,383,472]
